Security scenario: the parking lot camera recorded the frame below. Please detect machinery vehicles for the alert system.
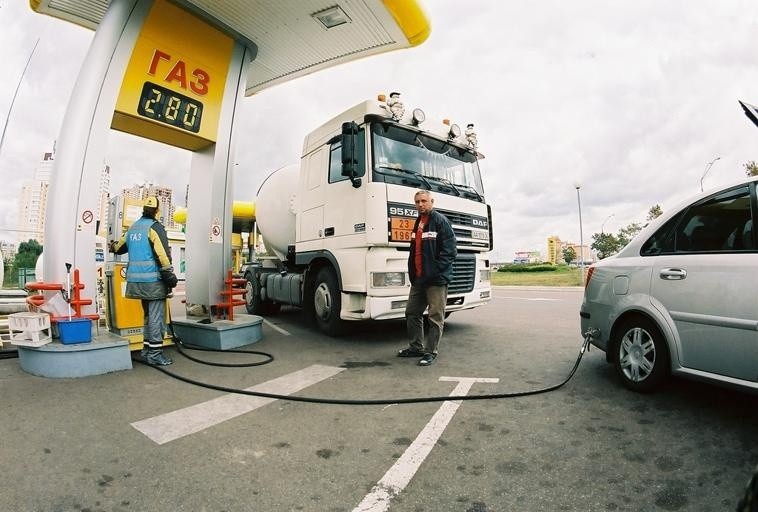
[242,91,499,333]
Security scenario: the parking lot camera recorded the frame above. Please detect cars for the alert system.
[578,180,758,395]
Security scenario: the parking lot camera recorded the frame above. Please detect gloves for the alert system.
[107,239,119,253]
[162,272,178,288]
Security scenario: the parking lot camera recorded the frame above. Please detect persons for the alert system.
[107,195,180,365]
[397,190,458,365]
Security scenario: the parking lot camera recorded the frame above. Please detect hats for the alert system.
[142,196,159,208]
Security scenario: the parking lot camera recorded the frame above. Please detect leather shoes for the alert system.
[398,348,437,366]
[140,347,173,367]
